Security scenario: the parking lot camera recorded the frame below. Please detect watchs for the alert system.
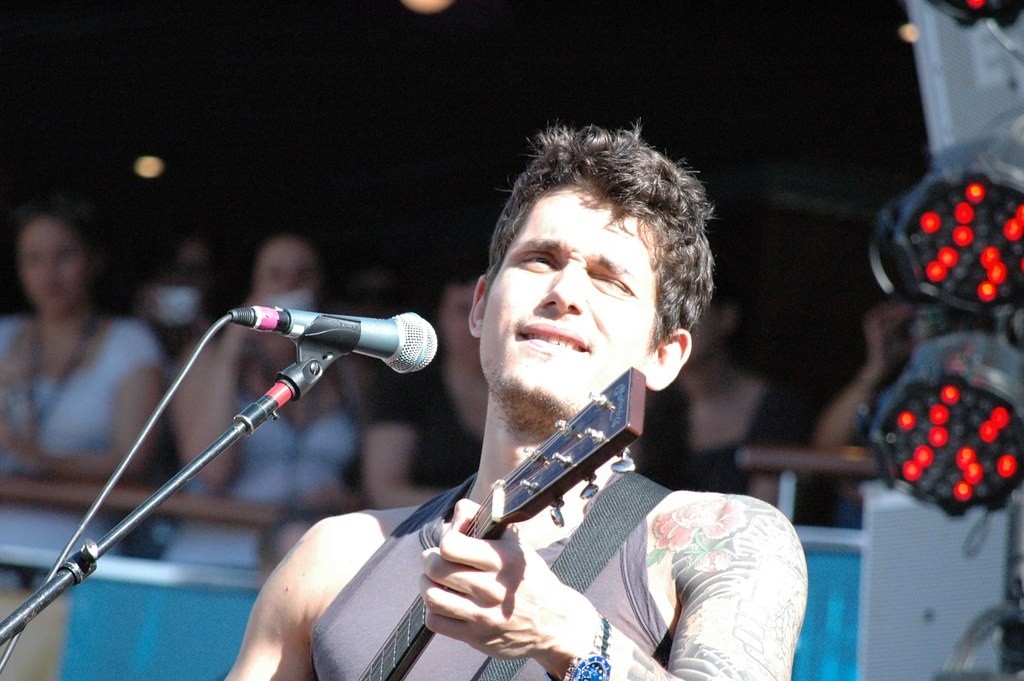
[542,607,613,681]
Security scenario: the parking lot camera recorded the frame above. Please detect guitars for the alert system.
[356,366,648,679]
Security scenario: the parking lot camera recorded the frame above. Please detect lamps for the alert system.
[869,330,1024,516]
[863,137,1024,334]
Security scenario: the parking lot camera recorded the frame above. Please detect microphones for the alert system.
[228,306,439,372]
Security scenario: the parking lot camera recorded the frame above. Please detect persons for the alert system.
[223,128,808,681]
[0,184,918,565]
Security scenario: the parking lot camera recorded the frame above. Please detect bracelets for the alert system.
[856,357,892,384]
